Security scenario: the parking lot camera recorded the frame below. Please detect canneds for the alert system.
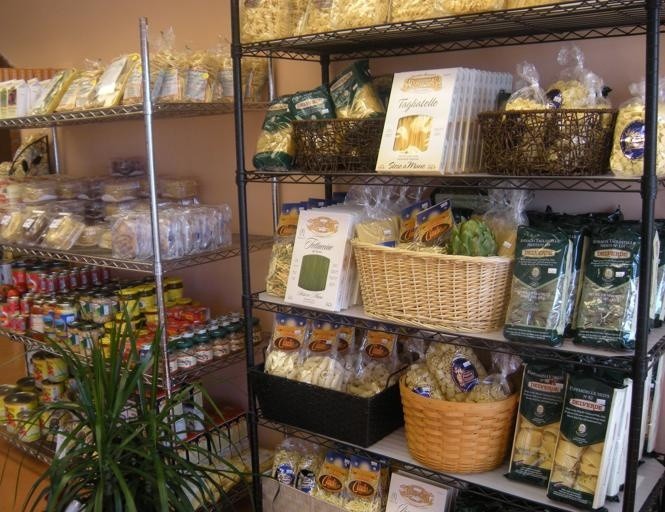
[0,253,262,376]
[0,349,249,478]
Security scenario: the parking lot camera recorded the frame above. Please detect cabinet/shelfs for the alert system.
[0,18,289,512]
[231,1,664,507]
